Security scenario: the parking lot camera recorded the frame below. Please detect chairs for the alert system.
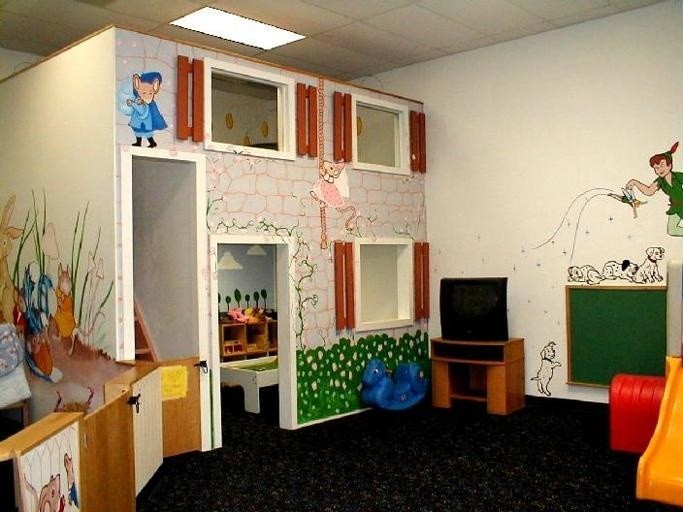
[1,323,30,428]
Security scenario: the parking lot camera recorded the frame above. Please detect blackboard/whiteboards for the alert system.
[565,285,668,388]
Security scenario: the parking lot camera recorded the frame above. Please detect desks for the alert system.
[220,356,278,415]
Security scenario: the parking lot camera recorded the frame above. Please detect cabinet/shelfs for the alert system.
[219,320,276,363]
[429,338,526,419]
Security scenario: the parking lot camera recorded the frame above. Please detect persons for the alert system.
[622,141,682,239]
[606,189,647,219]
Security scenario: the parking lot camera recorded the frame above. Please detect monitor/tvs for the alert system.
[439,277,509,342]
